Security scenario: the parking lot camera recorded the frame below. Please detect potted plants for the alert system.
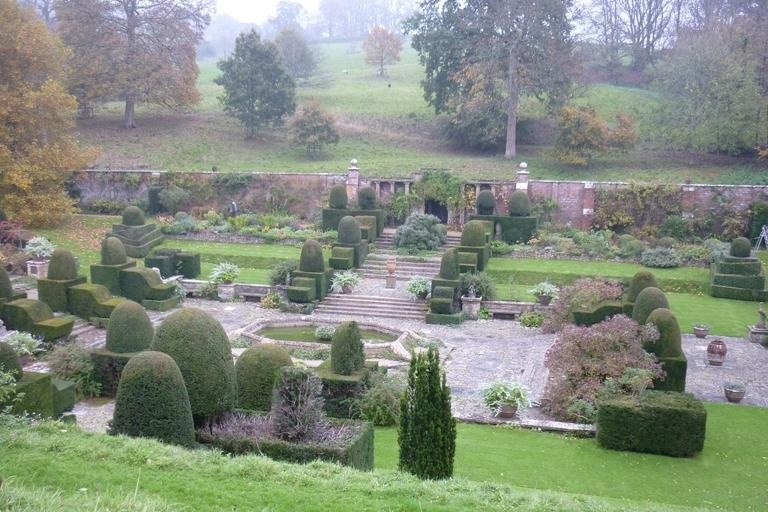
[22,235,56,280]
[482,380,542,422]
[329,268,362,294]
[693,322,746,403]
[460,286,482,321]
[523,282,560,305]
[405,275,431,300]
[209,262,239,301]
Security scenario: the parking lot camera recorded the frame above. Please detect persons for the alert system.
[230,199,237,217]
